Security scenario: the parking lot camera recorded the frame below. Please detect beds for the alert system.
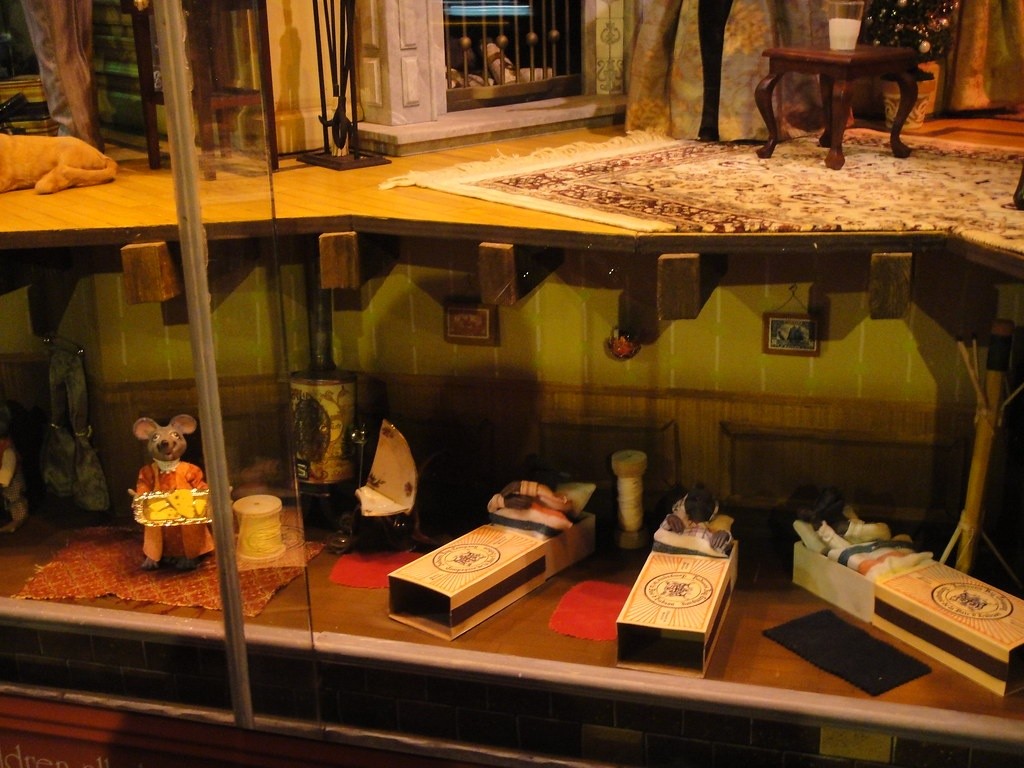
[791,488,1024,699]
[385,453,598,642]
[613,480,737,679]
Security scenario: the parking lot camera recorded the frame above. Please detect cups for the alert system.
[829,2,863,52]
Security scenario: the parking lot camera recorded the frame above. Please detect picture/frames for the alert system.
[443,303,499,347]
[763,312,821,357]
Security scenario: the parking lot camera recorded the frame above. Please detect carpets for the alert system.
[377,126,1024,257]
[10,526,327,617]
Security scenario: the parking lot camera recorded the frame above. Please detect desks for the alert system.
[754,44,918,170]
